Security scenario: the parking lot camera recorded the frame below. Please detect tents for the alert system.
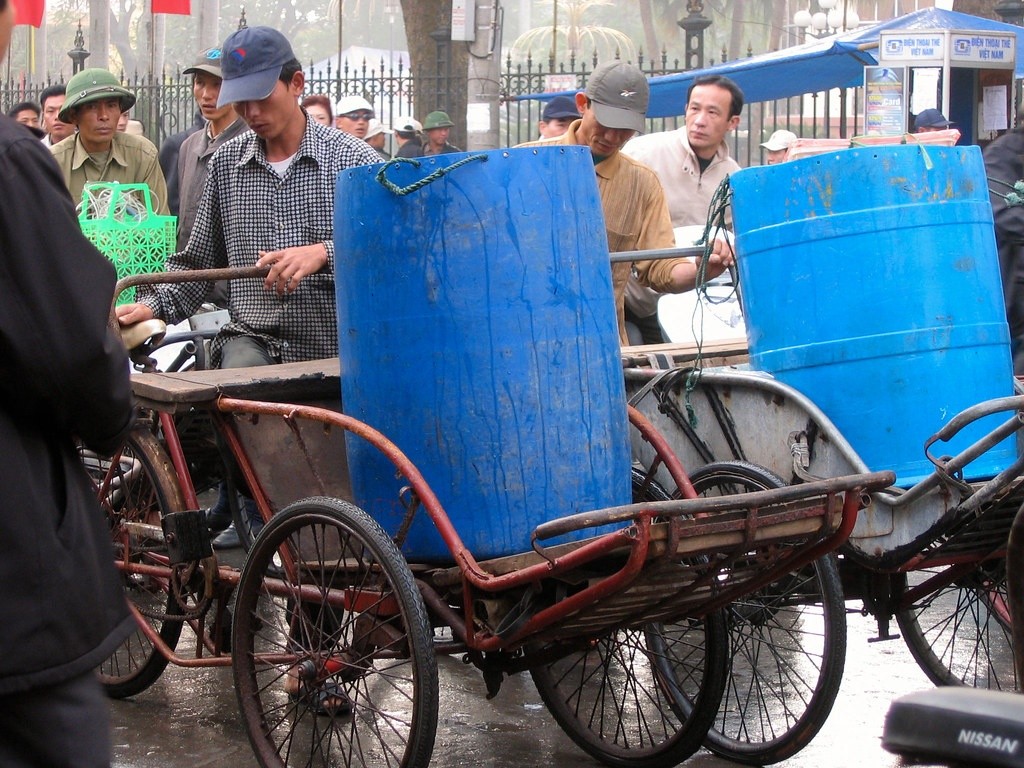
[496,6,1024,130]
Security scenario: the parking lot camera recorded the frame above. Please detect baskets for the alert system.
[77,181,178,307]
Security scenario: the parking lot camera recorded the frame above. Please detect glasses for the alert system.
[340,113,372,121]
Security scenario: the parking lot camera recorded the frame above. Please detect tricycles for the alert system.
[79,242,1024,768]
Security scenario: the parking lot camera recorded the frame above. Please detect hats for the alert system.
[337,96,374,116]
[586,59,649,135]
[58,68,137,124]
[215,25,294,108]
[393,117,415,132]
[915,108,957,130]
[544,97,581,118]
[183,46,222,78]
[422,111,455,130]
[759,130,796,151]
[363,118,394,140]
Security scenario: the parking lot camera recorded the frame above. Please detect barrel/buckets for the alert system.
[729,143,1019,489]
[332,146,633,562]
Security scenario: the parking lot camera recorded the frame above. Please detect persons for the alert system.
[115,25,387,717]
[9,85,463,497]
[539,97,585,140]
[759,130,797,165]
[509,62,735,345]
[44,68,175,585]
[914,108,955,133]
[983,102,1024,378]
[622,76,742,345]
[177,46,269,550]
[0,0,130,768]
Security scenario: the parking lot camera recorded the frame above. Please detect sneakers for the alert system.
[212,518,263,548]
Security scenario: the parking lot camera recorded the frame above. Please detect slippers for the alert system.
[289,681,351,716]
